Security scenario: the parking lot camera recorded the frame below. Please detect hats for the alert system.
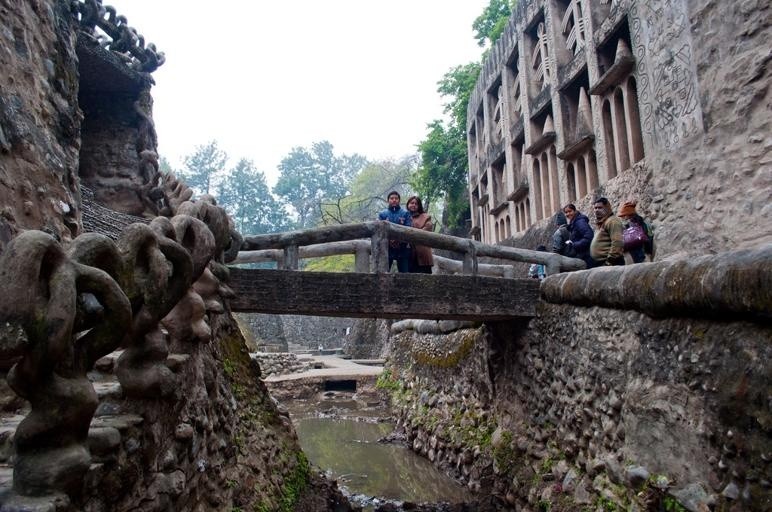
[618,202,636,217]
[555,212,567,225]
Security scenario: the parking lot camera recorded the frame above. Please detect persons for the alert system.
[552,211,571,272]
[378,191,408,272]
[527,245,546,280]
[562,203,594,268]
[589,197,625,266]
[616,201,653,263]
[406,195,434,273]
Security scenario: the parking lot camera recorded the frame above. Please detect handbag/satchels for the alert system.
[623,219,649,250]
